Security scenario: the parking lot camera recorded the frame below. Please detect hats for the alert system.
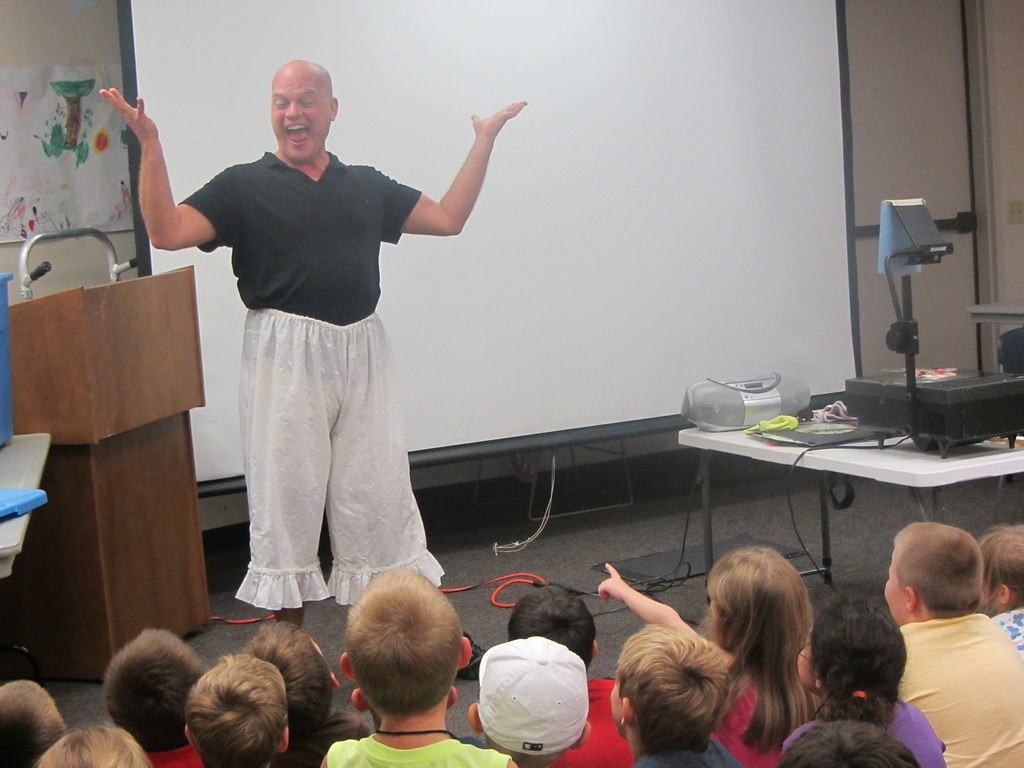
[478,635,590,757]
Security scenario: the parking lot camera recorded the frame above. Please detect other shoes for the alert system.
[455,633,488,679]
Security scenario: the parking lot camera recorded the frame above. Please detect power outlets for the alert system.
[1007,200,1024,224]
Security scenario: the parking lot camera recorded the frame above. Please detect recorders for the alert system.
[680,372,811,431]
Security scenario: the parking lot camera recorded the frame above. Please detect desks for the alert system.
[678,416,1024,605]
[966,299,1024,371]
[0,433,52,686]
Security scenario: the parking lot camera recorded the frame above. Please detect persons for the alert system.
[778,719,921,768]
[507,588,636,768]
[184,652,288,768]
[0,679,67,768]
[33,726,154,768]
[975,522,1024,658]
[97,59,528,628]
[610,622,746,768]
[468,635,590,768]
[240,620,371,768]
[781,592,947,768]
[884,522,1024,768]
[103,628,211,768]
[319,567,518,768]
[598,544,814,768]
